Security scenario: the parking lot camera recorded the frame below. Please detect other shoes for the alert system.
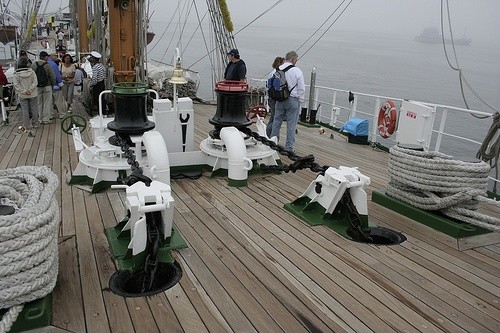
[24,129,31,131]
[31,123,38,127]
[68,104,72,110]
[42,119,53,124]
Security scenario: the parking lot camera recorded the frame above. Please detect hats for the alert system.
[90,50,102,59]
[39,50,51,57]
[226,48,239,54]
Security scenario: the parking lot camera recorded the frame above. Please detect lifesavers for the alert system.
[377,100,398,139]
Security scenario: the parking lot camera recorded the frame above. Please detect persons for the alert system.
[271,51,305,154]
[223,49,247,83]
[266,56,285,141]
[0,27,105,130]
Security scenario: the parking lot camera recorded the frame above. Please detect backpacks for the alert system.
[266,64,297,101]
[35,60,48,87]
